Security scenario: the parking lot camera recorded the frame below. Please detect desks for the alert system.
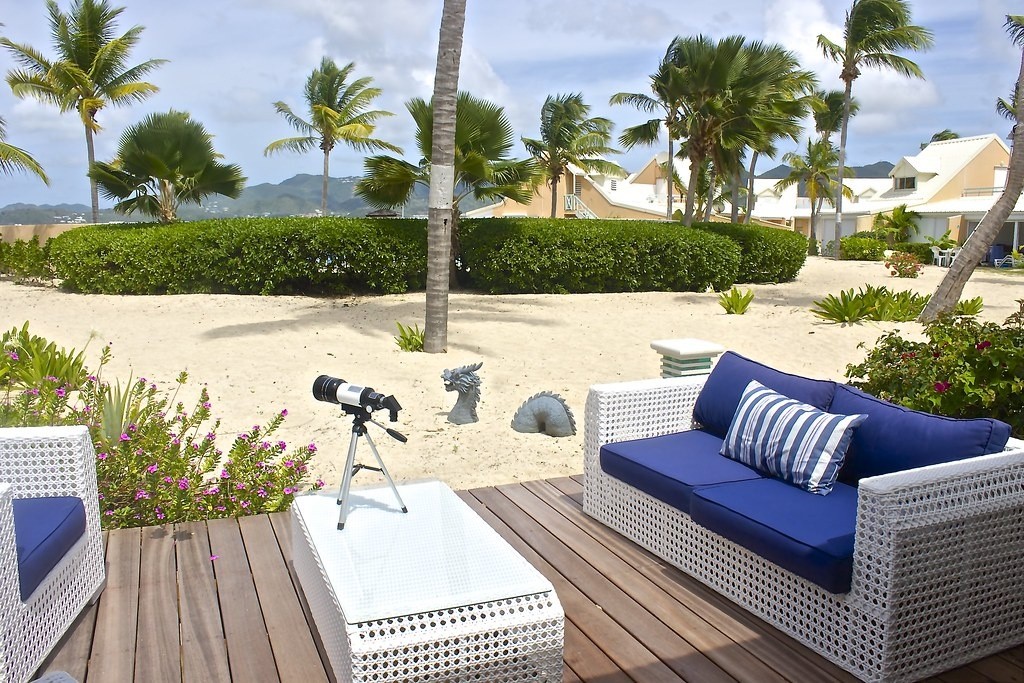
[941,249,955,267]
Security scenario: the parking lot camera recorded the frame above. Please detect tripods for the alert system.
[337,404,408,530]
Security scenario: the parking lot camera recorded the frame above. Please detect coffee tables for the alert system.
[288,478,563,683]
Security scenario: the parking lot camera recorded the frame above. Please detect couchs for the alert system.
[582,372,1024,683]
[0,426,107,683]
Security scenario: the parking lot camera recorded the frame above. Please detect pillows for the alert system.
[718,378,869,497]
[693,350,836,434]
[825,382,1010,489]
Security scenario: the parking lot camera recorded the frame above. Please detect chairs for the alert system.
[930,246,946,267]
[949,247,962,267]
